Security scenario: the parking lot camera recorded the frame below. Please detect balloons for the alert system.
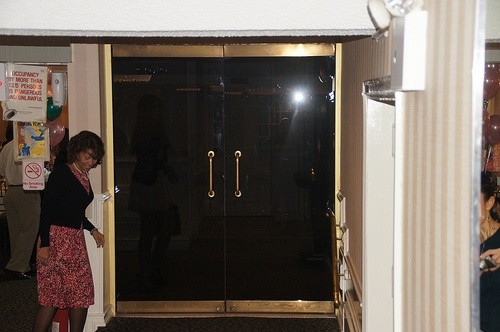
[48,121,65,147]
[47,97,62,121]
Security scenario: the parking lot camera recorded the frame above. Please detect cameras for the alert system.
[479,256,497,270]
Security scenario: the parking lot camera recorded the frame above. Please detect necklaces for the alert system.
[73,163,84,174]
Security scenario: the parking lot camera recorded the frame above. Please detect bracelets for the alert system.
[90,228,98,235]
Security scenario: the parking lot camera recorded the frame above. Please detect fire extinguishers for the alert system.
[50,309,68,332]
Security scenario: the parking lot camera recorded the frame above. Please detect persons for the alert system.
[0,139,42,280]
[479,172,500,332]
[33,129,105,332]
